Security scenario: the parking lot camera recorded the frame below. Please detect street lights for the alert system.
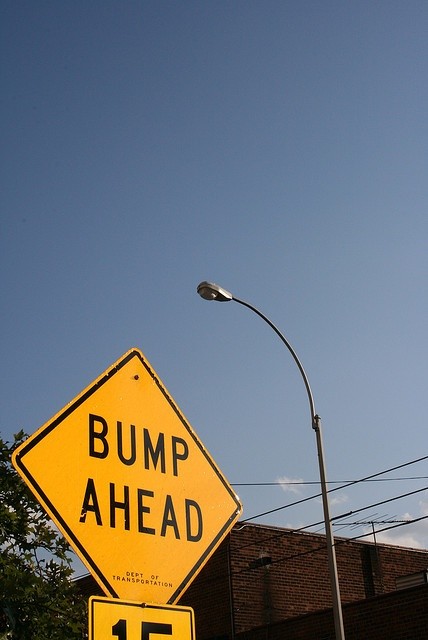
[198,282,347,639]
